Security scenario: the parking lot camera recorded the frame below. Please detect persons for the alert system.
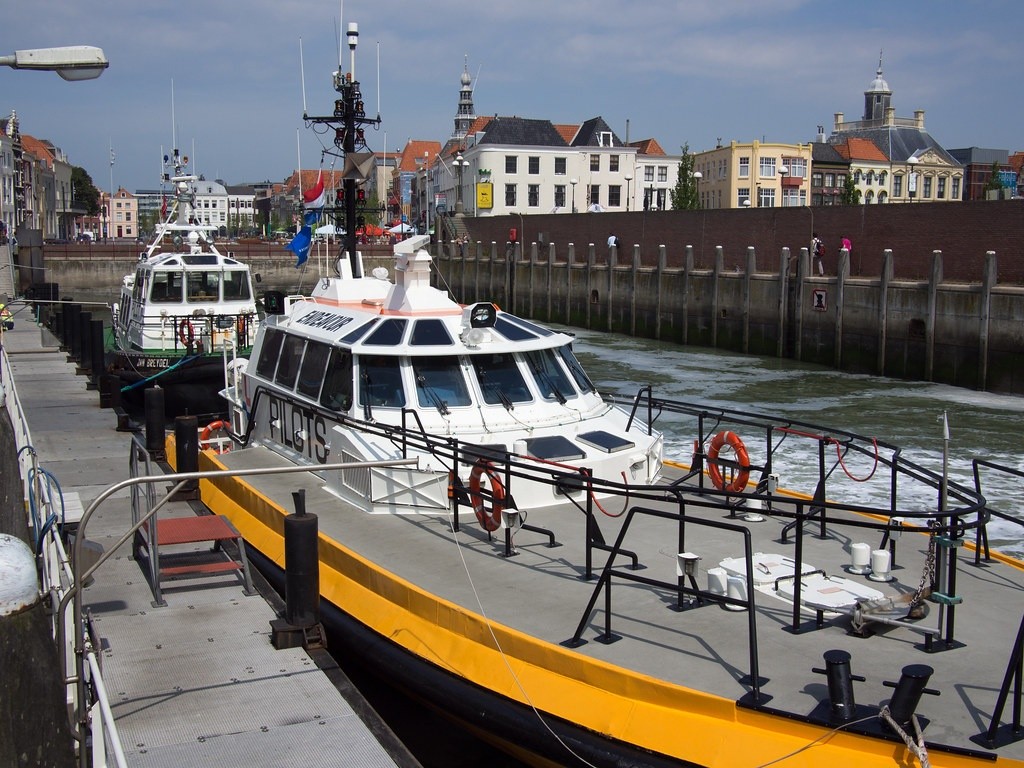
[838,234,852,254]
[509,228,517,246]
[0,304,14,331]
[809,233,824,277]
[463,232,468,241]
[455,235,463,256]
[607,232,618,249]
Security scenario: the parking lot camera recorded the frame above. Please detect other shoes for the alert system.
[819,274,823,277]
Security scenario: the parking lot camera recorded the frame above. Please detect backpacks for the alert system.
[813,238,825,257]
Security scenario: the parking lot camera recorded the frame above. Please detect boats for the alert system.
[167,0,1024,768]
[101,78,276,383]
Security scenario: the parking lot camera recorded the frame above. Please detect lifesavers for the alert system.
[180,320,194,345]
[200,421,234,456]
[468,459,504,532]
[708,430,750,493]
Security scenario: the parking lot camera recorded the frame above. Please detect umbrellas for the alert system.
[387,223,418,233]
[355,224,383,236]
[314,224,347,235]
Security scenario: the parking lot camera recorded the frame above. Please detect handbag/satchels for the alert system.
[838,245,842,251]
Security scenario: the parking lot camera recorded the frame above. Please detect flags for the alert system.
[284,225,311,267]
[161,195,167,216]
[303,170,325,225]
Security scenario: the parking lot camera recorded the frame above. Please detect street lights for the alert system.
[778,166,788,208]
[452,156,469,218]
[509,212,524,261]
[907,157,918,204]
[693,172,702,210]
[624,175,633,212]
[570,179,578,214]
